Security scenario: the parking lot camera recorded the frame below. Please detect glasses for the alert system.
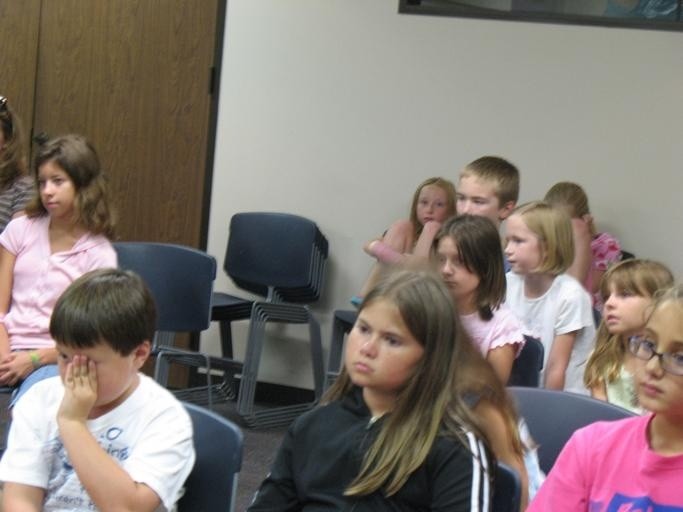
[629,335,683,377]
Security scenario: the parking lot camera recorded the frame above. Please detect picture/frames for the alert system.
[397,1,683,35]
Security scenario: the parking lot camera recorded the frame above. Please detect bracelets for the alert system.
[29,351,41,369]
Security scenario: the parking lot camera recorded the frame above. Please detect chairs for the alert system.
[506,335,546,389]
[505,384,641,478]
[176,398,246,512]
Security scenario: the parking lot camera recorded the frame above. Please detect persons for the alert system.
[440,350,546,509]
[0,134,119,418]
[585,259,678,417]
[521,288,683,511]
[0,268,197,512]
[0,96,40,236]
[353,155,623,395]
[232,272,492,511]
